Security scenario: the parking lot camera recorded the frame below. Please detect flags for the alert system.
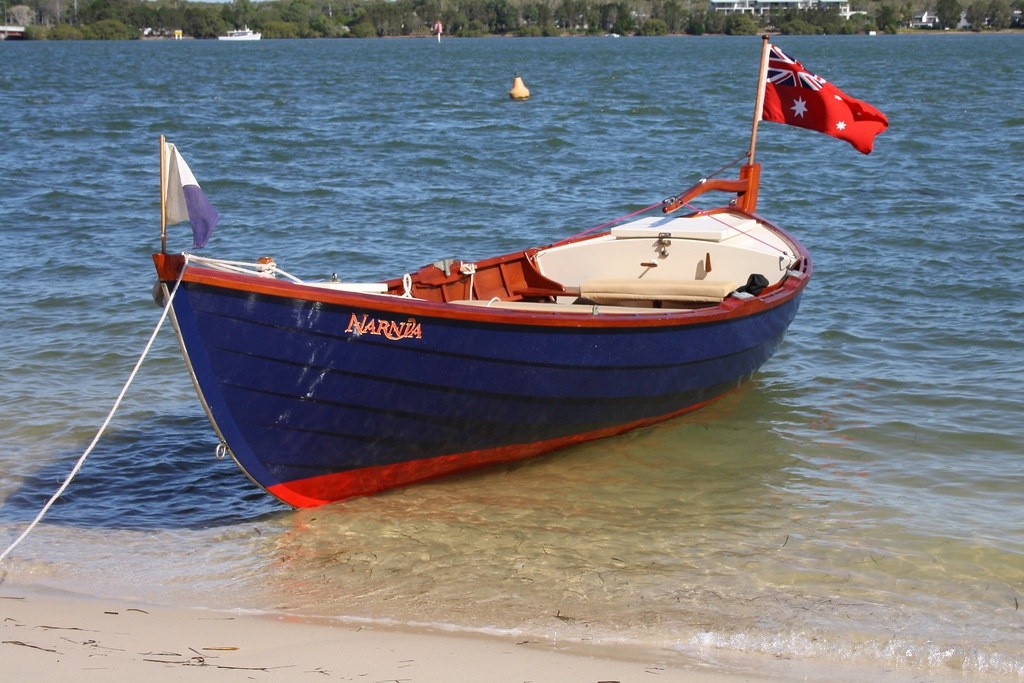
[758,44,888,155]
[161,137,219,247]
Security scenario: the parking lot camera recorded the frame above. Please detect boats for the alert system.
[153,163,814,510]
[0,25,25,40]
[218,23,262,40]
